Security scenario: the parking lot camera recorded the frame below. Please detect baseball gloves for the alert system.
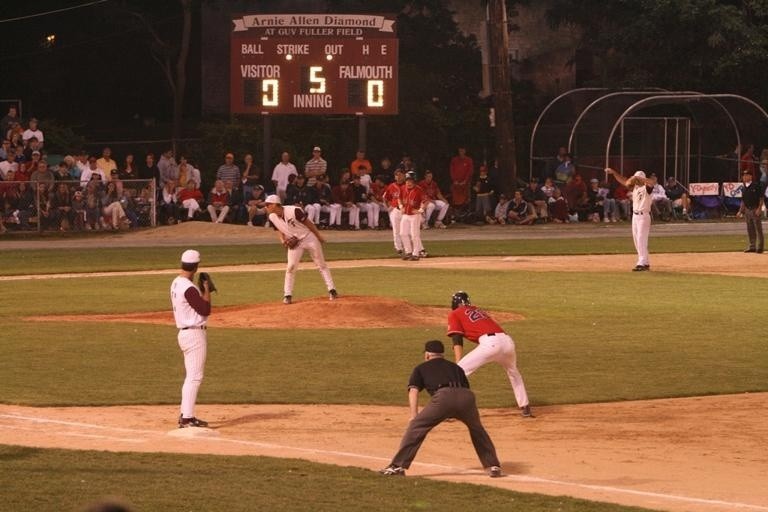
[284,236,298,249]
[198,273,217,294]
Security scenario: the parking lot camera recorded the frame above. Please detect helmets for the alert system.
[452,292,470,309]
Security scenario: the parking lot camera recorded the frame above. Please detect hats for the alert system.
[181,250,200,263]
[261,195,281,206]
[634,171,646,177]
[742,169,752,174]
[405,170,416,179]
[425,341,444,353]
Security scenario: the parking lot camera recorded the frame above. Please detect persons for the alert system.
[736,170,764,254]
[447,290,530,417]
[560,173,691,222]
[264,194,337,303]
[206,147,380,231]
[605,168,655,271]
[371,155,449,260]
[449,144,569,226]
[380,342,501,478]
[726,145,767,206]
[548,145,575,185]
[1,117,204,233]
[170,250,211,430]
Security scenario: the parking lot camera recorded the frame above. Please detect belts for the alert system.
[635,212,643,215]
[182,326,206,330]
[488,333,508,336]
[438,383,468,388]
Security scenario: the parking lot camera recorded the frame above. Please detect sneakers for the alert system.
[632,265,649,271]
[744,248,762,253]
[178,414,207,428]
[379,464,405,475]
[283,295,291,303]
[522,406,530,416]
[488,466,500,477]
[398,249,428,261]
[329,289,337,300]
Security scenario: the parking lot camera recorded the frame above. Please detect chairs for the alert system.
[671,206,694,221]
[696,195,721,219]
[722,198,742,217]
